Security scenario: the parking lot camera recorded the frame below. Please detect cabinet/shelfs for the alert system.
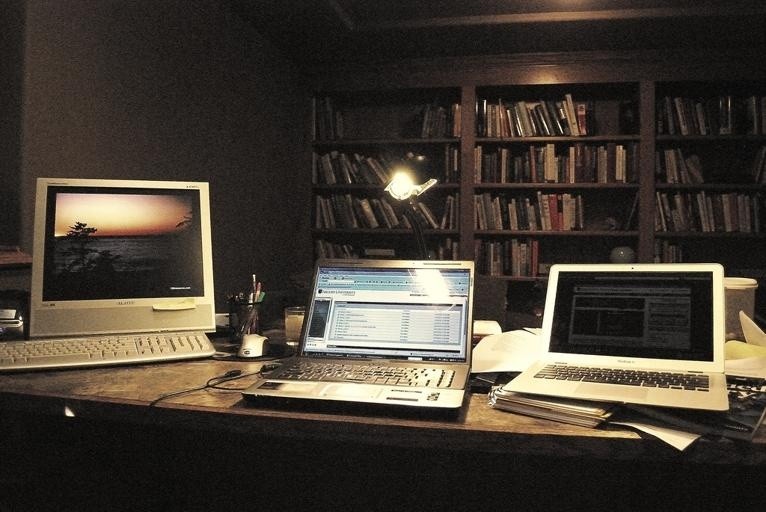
[310,51,766,330]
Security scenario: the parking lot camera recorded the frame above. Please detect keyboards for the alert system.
[0,329,216,373]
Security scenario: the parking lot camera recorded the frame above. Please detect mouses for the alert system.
[236,334,268,358]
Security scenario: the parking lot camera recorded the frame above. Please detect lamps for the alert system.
[384,172,438,261]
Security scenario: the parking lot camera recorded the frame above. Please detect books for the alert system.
[478,239,560,277]
[488,385,620,431]
[656,92,764,137]
[313,234,459,263]
[316,142,460,186]
[656,144,708,189]
[651,191,764,232]
[476,96,637,137]
[314,189,455,230]
[653,236,714,264]
[415,99,465,138]
[472,141,640,185]
[627,367,766,444]
[313,96,364,142]
[474,189,638,228]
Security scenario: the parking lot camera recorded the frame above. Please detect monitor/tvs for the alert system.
[28,178,218,337]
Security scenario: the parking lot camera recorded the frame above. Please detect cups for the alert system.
[284,307,306,346]
[725,276,759,344]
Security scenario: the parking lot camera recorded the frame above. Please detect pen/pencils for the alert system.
[248,293,254,335]
[252,274,260,335]
[242,291,266,338]
[252,282,262,334]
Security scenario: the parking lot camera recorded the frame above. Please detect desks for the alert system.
[0,329,766,512]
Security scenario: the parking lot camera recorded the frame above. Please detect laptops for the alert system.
[243,258,476,418]
[501,263,731,411]
